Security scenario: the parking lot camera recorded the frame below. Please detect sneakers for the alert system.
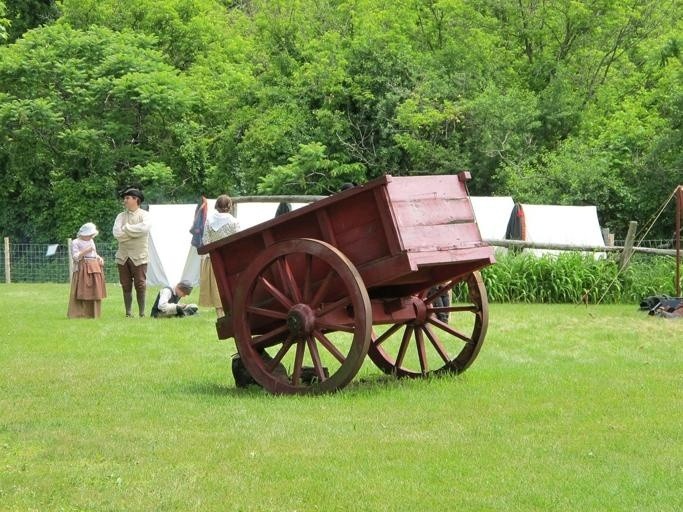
[176,306,185,316]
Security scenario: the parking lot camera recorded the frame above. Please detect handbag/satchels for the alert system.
[232,349,287,387]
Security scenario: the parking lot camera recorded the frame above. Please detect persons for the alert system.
[341,182,358,191]
[199,194,243,318]
[112,187,150,318]
[428,280,452,325]
[66,222,107,319]
[151,279,199,319]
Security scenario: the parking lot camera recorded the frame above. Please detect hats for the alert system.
[77,223,99,239]
[119,189,144,202]
